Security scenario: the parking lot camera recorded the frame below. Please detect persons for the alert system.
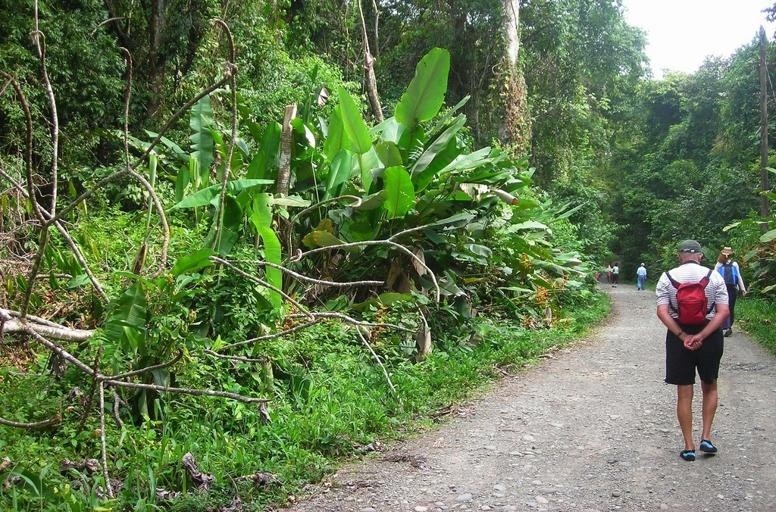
[714,246,746,337]
[656,239,730,461]
[636,262,647,291]
[607,263,619,288]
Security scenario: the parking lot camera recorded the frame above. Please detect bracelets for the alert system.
[676,329,683,337]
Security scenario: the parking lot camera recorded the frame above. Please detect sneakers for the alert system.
[680,449,696,461]
[700,439,717,452]
[722,328,732,337]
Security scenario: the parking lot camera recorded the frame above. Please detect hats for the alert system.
[721,246,734,259]
[677,240,701,252]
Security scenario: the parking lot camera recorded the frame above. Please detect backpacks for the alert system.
[717,261,738,287]
[664,270,715,326]
